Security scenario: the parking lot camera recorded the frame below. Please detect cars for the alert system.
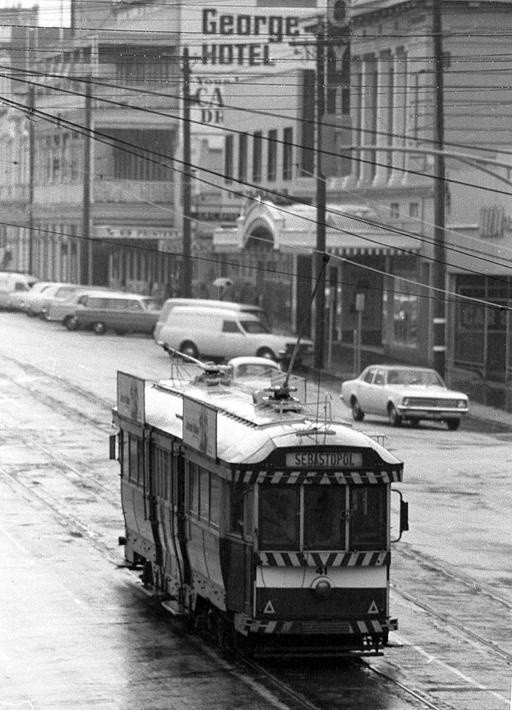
[340,363,470,431]
[0,269,161,336]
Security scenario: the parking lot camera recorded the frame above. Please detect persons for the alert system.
[220,281,234,302]
[198,284,209,298]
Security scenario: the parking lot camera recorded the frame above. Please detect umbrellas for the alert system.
[213,278,233,288]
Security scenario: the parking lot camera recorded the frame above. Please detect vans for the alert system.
[153,296,315,363]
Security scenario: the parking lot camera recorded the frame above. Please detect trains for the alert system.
[107,254,409,663]
